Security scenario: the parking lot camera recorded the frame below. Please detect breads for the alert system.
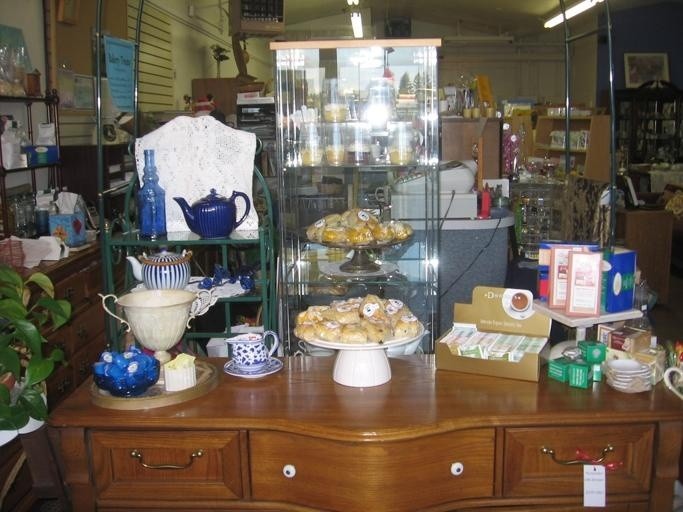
[306,207,415,246]
[287,269,348,297]
[294,293,423,345]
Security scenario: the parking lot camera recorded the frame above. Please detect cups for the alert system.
[463,108,495,119]
[438,100,449,113]
[225,330,279,372]
[298,78,424,168]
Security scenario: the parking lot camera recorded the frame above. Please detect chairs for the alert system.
[617,174,667,209]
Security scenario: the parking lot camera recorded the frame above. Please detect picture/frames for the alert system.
[624,52,670,88]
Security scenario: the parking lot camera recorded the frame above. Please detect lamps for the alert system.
[544,0,604,30]
[347,0,364,39]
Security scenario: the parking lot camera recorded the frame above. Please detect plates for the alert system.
[605,358,653,393]
[223,356,284,378]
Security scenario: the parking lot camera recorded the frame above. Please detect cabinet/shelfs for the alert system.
[102,160,273,356]
[533,115,610,182]
[9,234,127,414]
[441,114,500,188]
[268,38,443,355]
[47,353,683,512]
[615,210,673,306]
[0,87,63,236]
[614,79,683,188]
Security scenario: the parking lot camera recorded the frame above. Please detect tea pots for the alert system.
[126,248,192,291]
[172,188,251,240]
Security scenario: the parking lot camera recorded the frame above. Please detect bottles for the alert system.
[137,150,167,241]
[7,193,37,238]
